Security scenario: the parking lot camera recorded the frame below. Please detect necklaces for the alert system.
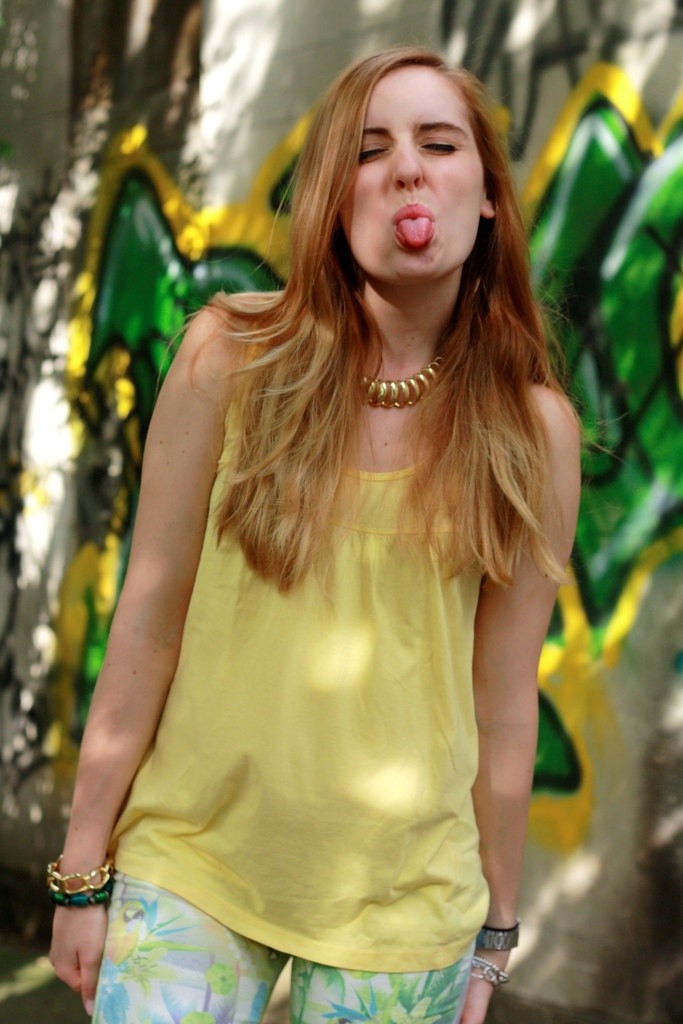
[356,354,444,408]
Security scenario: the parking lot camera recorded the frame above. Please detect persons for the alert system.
[47,46,584,1024]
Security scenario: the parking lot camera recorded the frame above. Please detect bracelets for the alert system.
[477,921,519,950]
[470,956,508,988]
[47,857,115,906]
